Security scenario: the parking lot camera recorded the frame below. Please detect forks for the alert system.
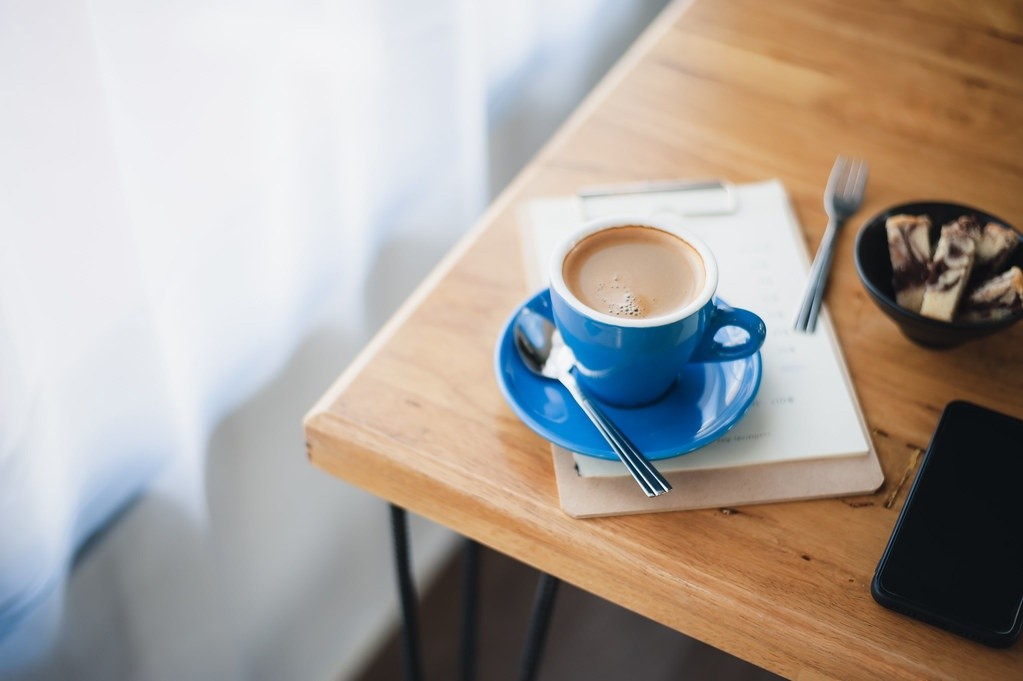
[791,153,867,332]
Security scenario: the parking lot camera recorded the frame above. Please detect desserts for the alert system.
[886,210,1023,324]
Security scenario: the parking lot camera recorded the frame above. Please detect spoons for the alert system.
[515,311,673,497]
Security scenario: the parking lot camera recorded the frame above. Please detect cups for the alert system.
[549,212,767,406]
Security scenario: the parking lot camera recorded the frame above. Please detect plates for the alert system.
[495,285,763,462]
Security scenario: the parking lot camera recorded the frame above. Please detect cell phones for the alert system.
[870,398,1023,650]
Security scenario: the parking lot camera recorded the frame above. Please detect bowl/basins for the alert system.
[854,199,1023,354]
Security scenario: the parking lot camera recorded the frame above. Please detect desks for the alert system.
[303,0,1023,681]
